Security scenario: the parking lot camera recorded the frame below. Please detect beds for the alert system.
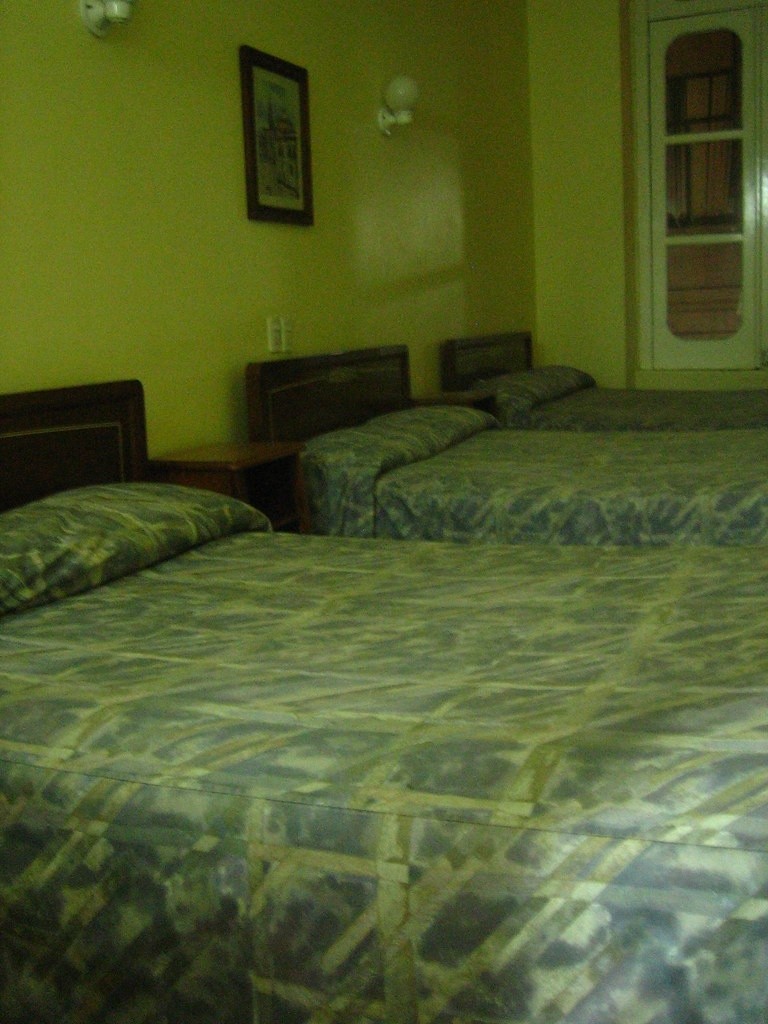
[438,329,768,429]
[0,380,768,1024]
[240,343,768,545]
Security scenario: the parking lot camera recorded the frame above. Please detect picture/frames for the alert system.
[240,44,314,224]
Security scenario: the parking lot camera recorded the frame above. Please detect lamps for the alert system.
[379,75,420,137]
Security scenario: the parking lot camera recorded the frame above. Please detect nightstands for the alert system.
[416,389,498,418]
[150,440,313,533]
